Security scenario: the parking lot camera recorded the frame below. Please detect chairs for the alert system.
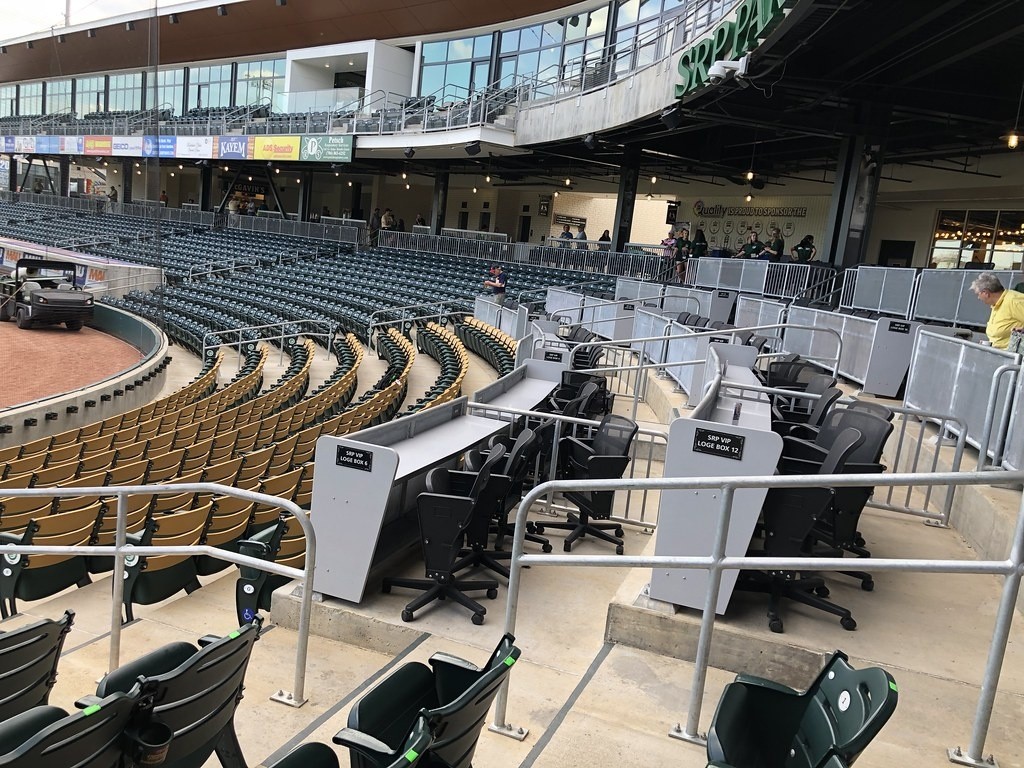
[0,200,715,358]
[383,326,641,626]
[965,262,996,270]
[679,312,895,634]
[779,297,946,326]
[1,315,521,768]
[0,62,618,135]
[704,649,898,768]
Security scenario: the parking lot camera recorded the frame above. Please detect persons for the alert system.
[107,186,117,212]
[480,224,500,240]
[160,190,168,218]
[790,235,817,264]
[415,213,426,226]
[597,230,611,273]
[759,228,784,263]
[575,223,587,249]
[485,265,507,304]
[660,230,676,256]
[670,228,691,273]
[689,229,708,258]
[558,224,574,248]
[969,272,1024,350]
[731,231,766,259]
[32,178,44,194]
[369,208,406,248]
[229,195,268,221]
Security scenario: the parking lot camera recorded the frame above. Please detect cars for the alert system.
[0,258,95,332]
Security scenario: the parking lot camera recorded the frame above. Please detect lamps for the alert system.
[464,141,482,156]
[644,183,654,201]
[88,29,96,38]
[168,14,179,24]
[585,132,598,150]
[217,5,227,16]
[557,13,592,27]
[744,184,755,202]
[404,147,415,158]
[275,0,286,6]
[0,47,7,54]
[27,41,33,49]
[741,126,760,181]
[659,103,684,130]
[57,35,65,43]
[999,84,1024,149]
[126,22,135,31]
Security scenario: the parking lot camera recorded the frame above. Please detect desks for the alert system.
[310,278,571,605]
[616,256,1023,492]
[546,288,785,614]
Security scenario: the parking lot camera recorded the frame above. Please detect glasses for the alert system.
[669,233,672,235]
